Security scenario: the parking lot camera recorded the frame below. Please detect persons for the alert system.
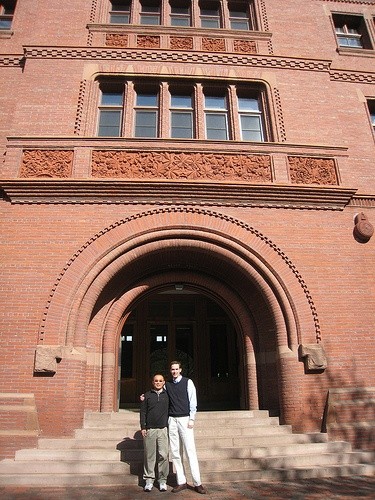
[139,360,208,494]
[140,374,168,491]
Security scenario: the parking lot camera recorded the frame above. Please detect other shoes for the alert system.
[195,484,208,494]
[171,482,188,493]
[159,483,168,491]
[144,483,154,491]
